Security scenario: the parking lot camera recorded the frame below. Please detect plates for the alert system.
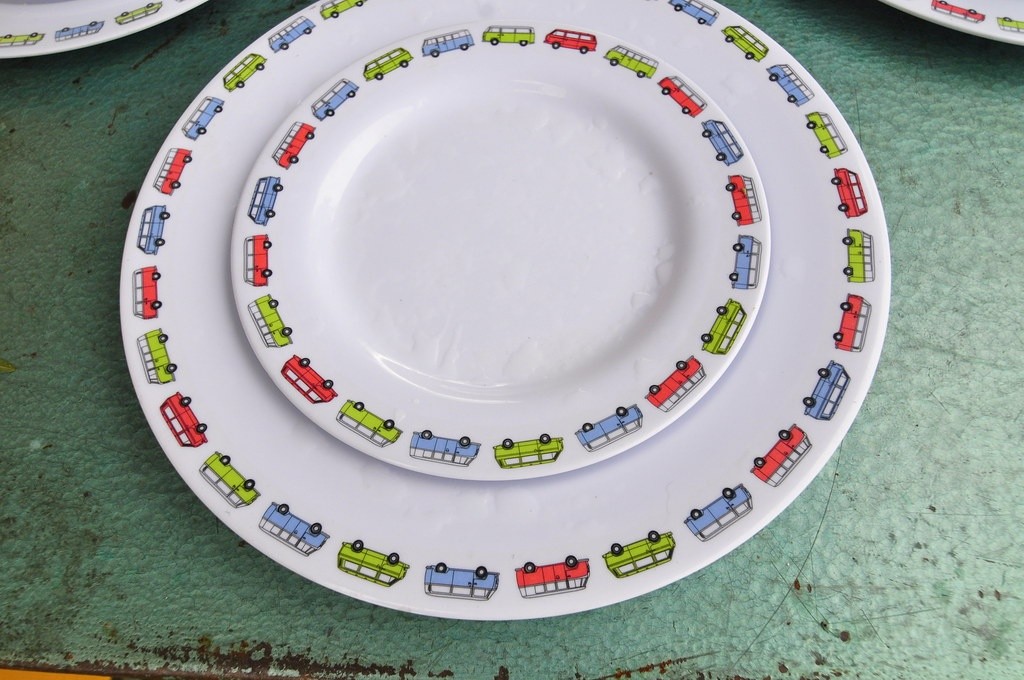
[881,0,1024,54]
[120,0,891,621]
[230,20,770,484]
[1,0,210,59]
